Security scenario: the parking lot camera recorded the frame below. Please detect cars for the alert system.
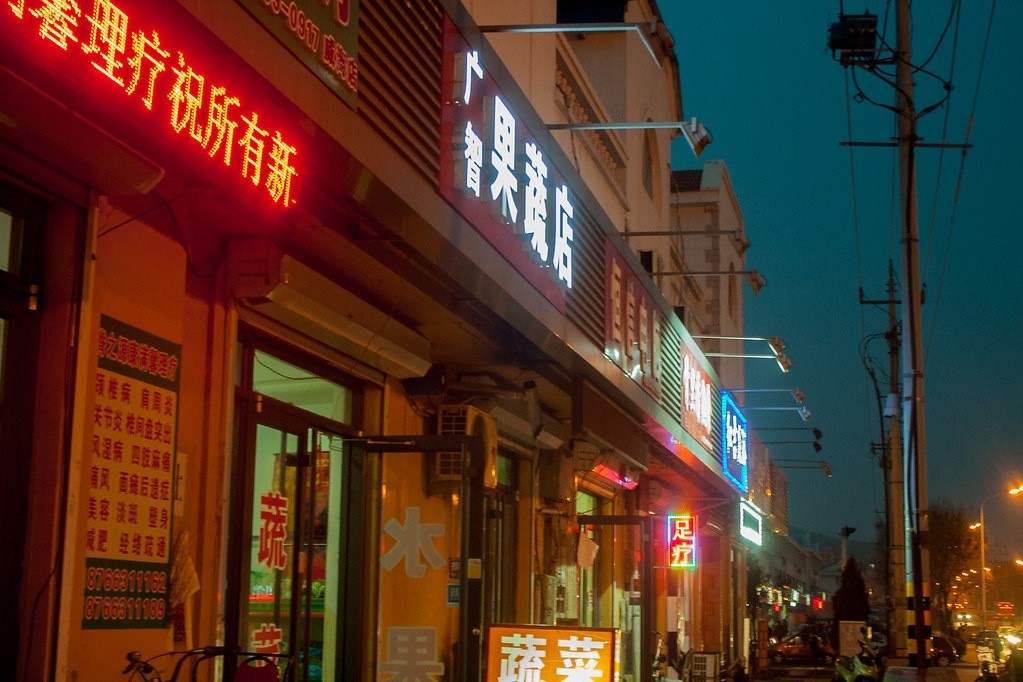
[932,624,1023,682]
[770,623,836,666]
[870,631,887,651]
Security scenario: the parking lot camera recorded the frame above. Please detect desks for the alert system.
[247,610,323,618]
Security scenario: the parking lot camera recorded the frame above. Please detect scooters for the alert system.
[834,626,883,682]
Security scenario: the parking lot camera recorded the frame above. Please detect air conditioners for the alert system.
[693,654,720,682]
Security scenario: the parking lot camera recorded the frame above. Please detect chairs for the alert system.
[192,651,294,682]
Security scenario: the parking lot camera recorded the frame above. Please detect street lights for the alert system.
[980,486,1023,629]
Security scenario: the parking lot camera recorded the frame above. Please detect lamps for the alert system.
[478,15,675,68]
[760,442,822,454]
[618,228,750,258]
[738,407,810,422]
[704,353,792,373]
[545,116,709,158]
[651,272,767,295]
[728,388,806,404]
[437,404,497,489]
[691,336,786,357]
[772,459,833,480]
[752,427,823,440]
